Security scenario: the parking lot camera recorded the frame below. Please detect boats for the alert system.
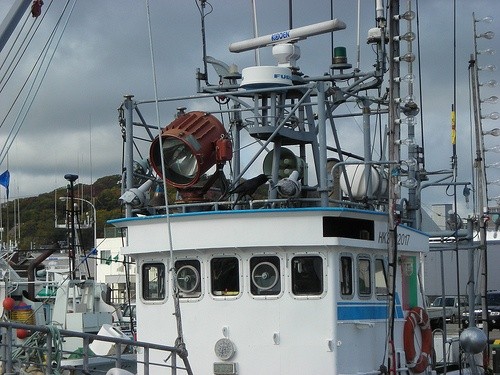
[0,1,496,375]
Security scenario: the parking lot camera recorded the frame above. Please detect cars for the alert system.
[431,294,468,324]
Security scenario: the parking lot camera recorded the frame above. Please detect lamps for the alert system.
[487,179,500,203]
[476,31,494,39]
[398,178,417,189]
[477,48,496,56]
[399,157,417,169]
[477,64,496,73]
[475,16,495,25]
[394,73,416,84]
[482,128,500,137]
[393,11,415,21]
[394,115,417,126]
[484,145,500,153]
[393,32,416,42]
[394,95,416,106]
[394,52,415,63]
[481,112,500,120]
[480,96,498,105]
[479,79,497,88]
[485,162,500,169]
[394,136,416,148]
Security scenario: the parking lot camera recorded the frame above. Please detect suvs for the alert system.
[462,290,500,329]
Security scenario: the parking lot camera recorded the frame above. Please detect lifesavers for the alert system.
[403,307,432,373]
